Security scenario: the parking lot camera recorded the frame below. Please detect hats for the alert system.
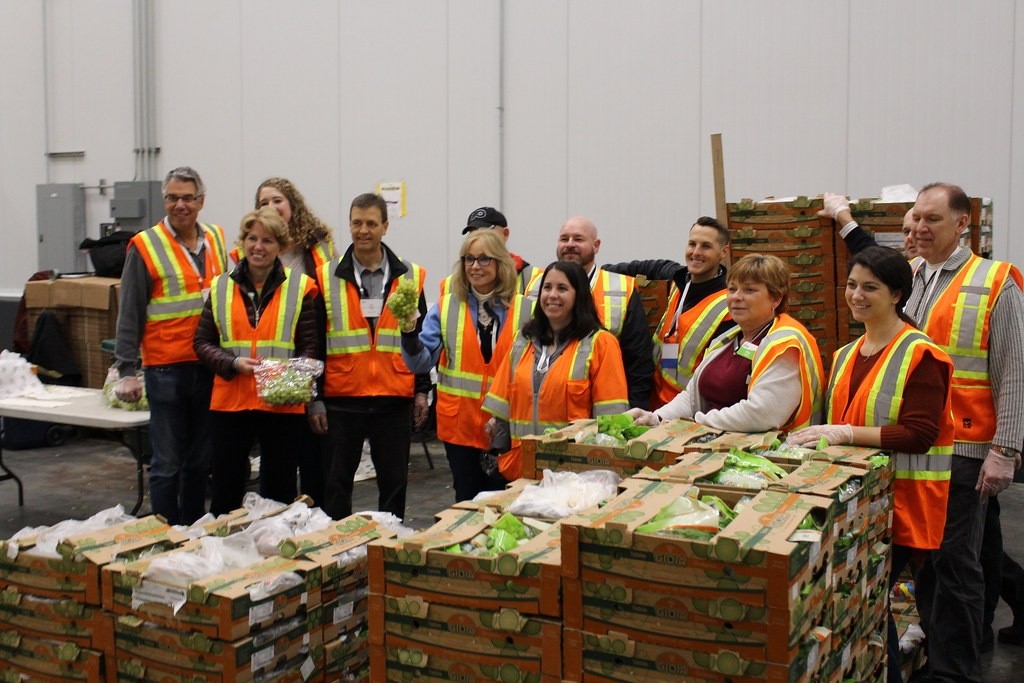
[462,207,507,234]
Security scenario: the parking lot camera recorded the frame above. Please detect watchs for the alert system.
[992,444,1016,457]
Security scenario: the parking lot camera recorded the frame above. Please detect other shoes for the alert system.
[998,611,1022,646]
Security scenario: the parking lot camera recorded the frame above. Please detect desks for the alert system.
[0,382,151,517]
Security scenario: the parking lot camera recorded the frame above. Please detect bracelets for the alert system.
[233,356,239,370]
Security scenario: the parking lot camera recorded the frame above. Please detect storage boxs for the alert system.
[0,587,99,651]
[726,193,831,228]
[835,256,912,287]
[102,539,322,641]
[369,414,894,683]
[324,622,370,679]
[731,250,835,285]
[225,494,397,604]
[0,630,101,679]
[0,512,176,605]
[0,659,88,683]
[790,310,837,337]
[836,227,992,258]
[839,322,866,348]
[787,283,837,311]
[836,196,995,227]
[321,585,369,645]
[836,287,866,323]
[92,604,323,683]
[728,229,836,256]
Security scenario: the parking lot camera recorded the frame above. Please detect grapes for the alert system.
[104,381,149,410]
[262,369,312,406]
[387,278,418,330]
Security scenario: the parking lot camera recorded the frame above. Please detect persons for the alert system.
[623,253,825,436]
[601,216,739,412]
[399,227,537,504]
[817,192,1024,653]
[480,261,630,482]
[524,217,655,410]
[305,193,433,524]
[786,245,955,683]
[113,166,230,526]
[227,178,338,276]
[903,182,1024,683]
[438,206,547,304]
[193,206,325,515]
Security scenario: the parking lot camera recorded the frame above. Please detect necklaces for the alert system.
[477,312,490,322]
[863,318,899,363]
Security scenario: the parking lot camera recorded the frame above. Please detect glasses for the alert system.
[461,254,494,267]
[165,193,200,203]
